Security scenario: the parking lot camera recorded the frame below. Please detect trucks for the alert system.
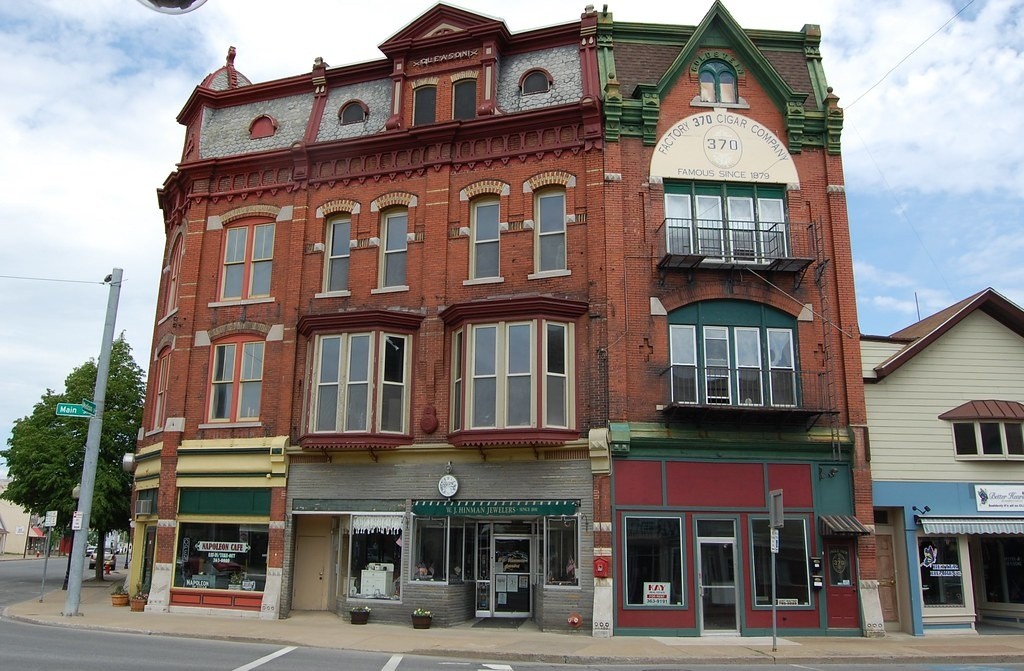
[649,543,784,616]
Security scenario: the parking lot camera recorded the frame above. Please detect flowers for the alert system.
[414,608,432,617]
[131,591,149,600]
[111,585,128,595]
[352,606,372,612]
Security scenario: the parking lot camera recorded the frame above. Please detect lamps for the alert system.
[912,506,931,515]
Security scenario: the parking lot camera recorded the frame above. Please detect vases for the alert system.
[349,611,369,624]
[129,599,148,612]
[110,593,129,607]
[411,614,432,629]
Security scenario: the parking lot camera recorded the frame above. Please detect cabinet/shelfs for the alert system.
[360,563,394,595]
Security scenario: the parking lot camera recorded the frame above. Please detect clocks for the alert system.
[438,475,459,497]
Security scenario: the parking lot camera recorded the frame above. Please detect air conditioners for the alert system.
[134,500,152,515]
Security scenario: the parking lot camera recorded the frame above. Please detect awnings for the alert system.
[914,515,1024,536]
[818,515,871,536]
[29,528,46,537]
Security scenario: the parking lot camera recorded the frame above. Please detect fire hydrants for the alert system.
[104,564,112,576]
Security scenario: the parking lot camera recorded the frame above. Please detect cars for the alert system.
[181,554,242,587]
[90,547,115,570]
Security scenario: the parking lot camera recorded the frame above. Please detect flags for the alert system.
[567,557,575,574]
